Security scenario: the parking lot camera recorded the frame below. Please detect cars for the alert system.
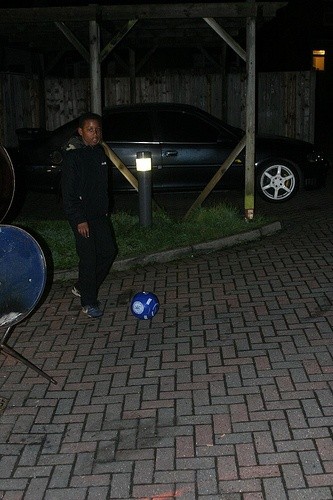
[18,101,324,203]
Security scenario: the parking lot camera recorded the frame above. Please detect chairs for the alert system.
[1,223,61,384]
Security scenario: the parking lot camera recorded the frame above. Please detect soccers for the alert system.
[132,291,159,319]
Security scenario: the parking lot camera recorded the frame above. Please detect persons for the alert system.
[60,112,118,319]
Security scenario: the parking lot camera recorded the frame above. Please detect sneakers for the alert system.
[71,285,81,297]
[82,305,103,317]
